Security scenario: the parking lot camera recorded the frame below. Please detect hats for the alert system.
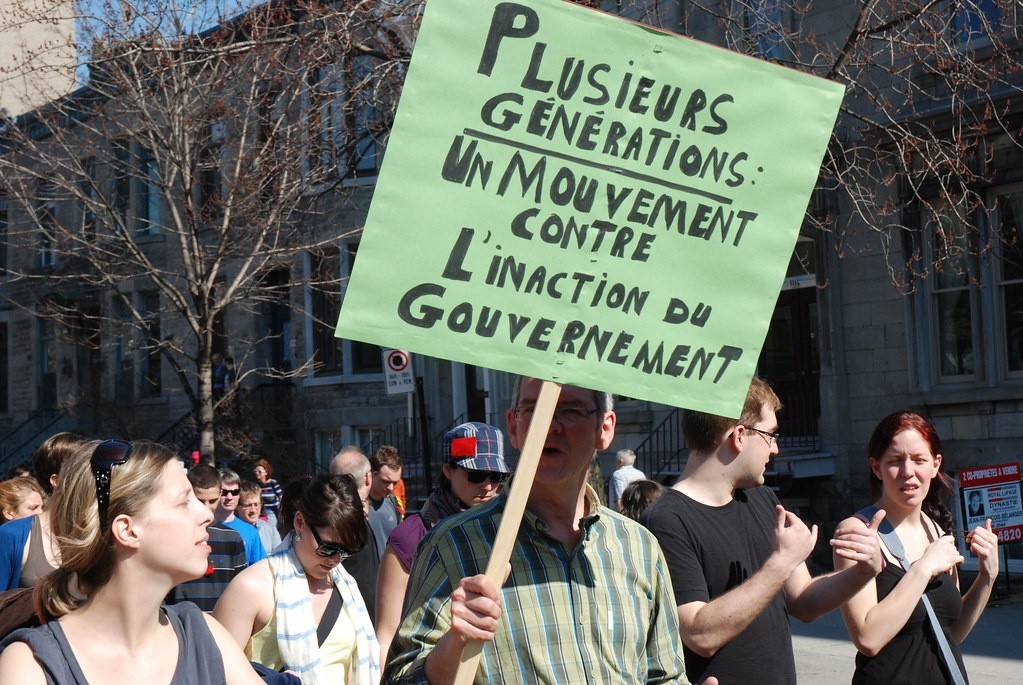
[442,421,511,474]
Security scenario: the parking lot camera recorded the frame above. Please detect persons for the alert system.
[212,353,240,412]
[0,432,82,642]
[833,411,999,685]
[968,491,985,517]
[160,446,407,616]
[213,474,381,685]
[375,423,511,678]
[0,439,268,685]
[637,377,885,685]
[378,376,717,685]
[587,450,664,522]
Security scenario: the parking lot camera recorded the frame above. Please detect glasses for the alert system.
[238,502,263,509]
[221,488,241,496]
[728,425,779,444]
[90,438,132,535]
[513,406,605,425]
[456,464,506,483]
[305,519,354,559]
